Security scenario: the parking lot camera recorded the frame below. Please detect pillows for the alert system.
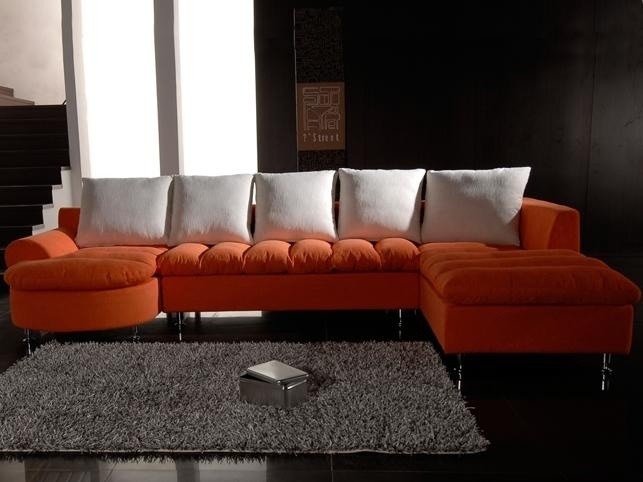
[336,164,428,246]
[252,168,339,243]
[170,172,257,248]
[425,166,530,246]
[75,173,174,247]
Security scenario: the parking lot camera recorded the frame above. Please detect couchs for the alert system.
[4,204,643,394]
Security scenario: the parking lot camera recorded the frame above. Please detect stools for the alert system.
[2,259,162,357]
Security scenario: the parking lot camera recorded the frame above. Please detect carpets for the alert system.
[0,339,492,457]
